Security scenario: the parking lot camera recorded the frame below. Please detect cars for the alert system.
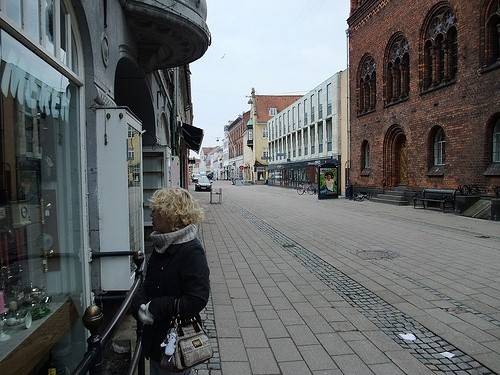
[191,174,201,182]
[194,176,213,192]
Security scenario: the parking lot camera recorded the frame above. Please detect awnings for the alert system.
[181,121,203,153]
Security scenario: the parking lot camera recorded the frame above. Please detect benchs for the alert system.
[412,188,458,213]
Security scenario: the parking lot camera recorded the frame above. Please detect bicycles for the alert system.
[296,179,316,195]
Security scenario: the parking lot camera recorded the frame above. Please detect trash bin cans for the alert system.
[210,187,222,204]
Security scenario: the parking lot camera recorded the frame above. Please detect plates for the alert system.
[14,306,51,321]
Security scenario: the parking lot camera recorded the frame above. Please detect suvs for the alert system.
[199,171,209,179]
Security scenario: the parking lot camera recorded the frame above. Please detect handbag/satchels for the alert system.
[161,314,214,375]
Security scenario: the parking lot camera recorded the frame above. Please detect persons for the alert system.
[128,188,210,375]
[320,174,337,191]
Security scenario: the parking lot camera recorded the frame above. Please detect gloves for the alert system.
[144,302,153,319]
[138,304,153,325]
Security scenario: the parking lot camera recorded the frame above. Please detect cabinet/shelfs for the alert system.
[143,152,164,208]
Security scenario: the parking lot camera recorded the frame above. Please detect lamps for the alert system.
[168,68,192,75]
